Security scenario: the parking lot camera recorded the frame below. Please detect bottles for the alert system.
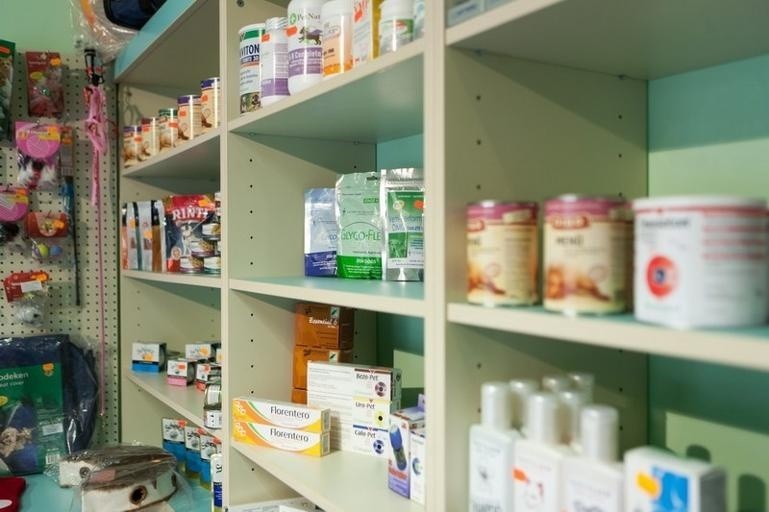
[464,366,625,510]
[239,0,432,116]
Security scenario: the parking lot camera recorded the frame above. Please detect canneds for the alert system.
[237,22,264,116]
[542,194,635,314]
[632,197,765,330]
[141,117,160,162]
[200,77,222,134]
[158,108,179,153]
[466,199,542,307]
[123,126,142,167]
[178,94,203,145]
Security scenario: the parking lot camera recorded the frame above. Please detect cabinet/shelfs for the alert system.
[111,0,769,512]
